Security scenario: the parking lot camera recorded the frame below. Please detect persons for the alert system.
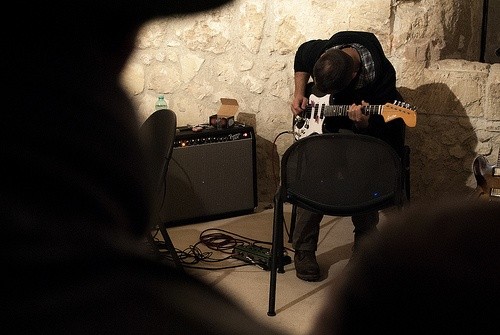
[317,202,499,334]
[292,32,398,282]
[0,0,278,335]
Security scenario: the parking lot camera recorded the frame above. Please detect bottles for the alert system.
[155,96,167,111]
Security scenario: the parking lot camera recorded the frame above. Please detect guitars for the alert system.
[293,94,417,141]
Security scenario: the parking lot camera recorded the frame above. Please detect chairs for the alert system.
[264,131,415,320]
[105,109,184,271]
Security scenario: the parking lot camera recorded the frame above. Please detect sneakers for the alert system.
[293,251,320,281]
